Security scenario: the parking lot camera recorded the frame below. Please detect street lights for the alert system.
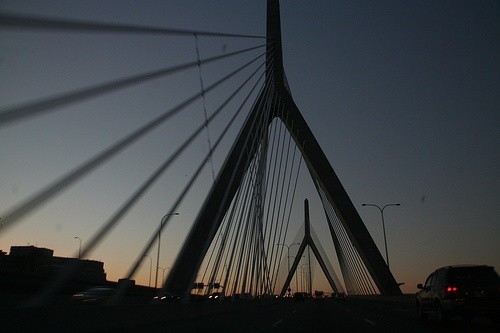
[361,202,401,268]
[154,213,180,289]
[276,243,300,297]
[75,237,81,259]
[145,255,152,287]
[158,266,170,288]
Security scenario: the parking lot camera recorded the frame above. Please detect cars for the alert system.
[272,292,312,304]
[415,265,500,329]
[80,285,117,305]
[208,292,226,305]
[152,293,183,305]
